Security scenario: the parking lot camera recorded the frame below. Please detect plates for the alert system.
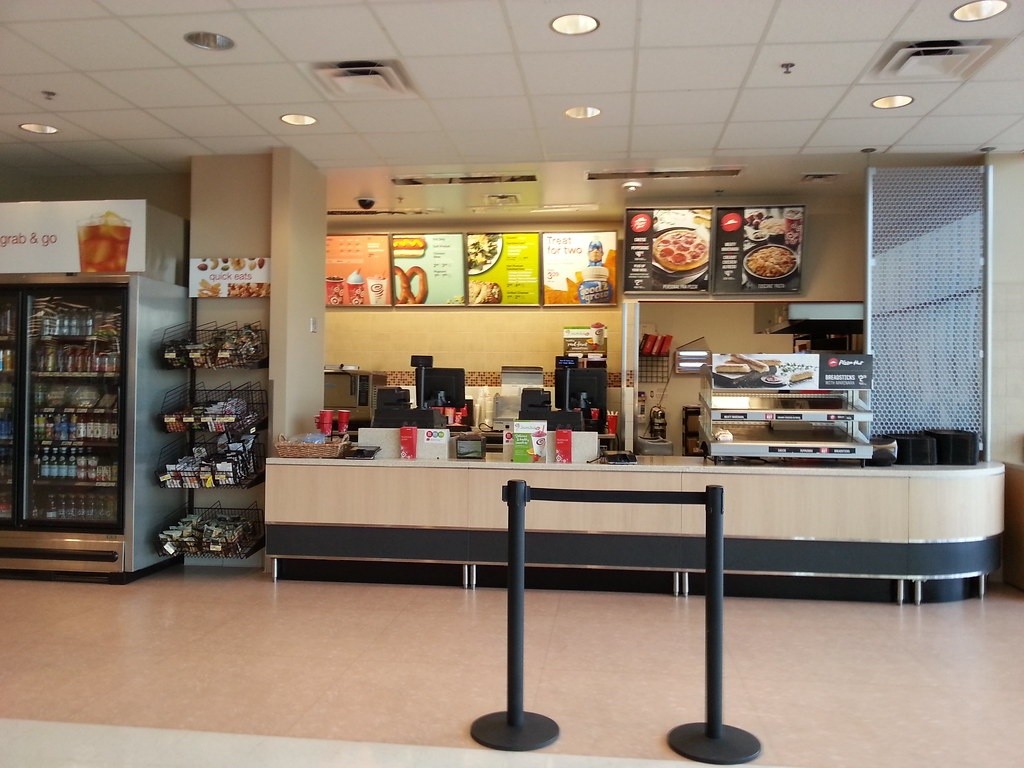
[468,236,504,276]
[743,242,798,281]
[748,229,770,241]
[758,218,785,237]
[760,373,788,386]
[652,226,709,277]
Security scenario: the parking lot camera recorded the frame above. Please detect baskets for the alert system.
[157,379,269,433]
[152,434,266,491]
[274,434,350,459]
[156,320,268,369]
[153,500,265,560]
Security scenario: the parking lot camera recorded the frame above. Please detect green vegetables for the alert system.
[467,234,499,271]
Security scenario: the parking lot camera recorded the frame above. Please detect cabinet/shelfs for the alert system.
[154,320,270,558]
[698,363,874,468]
[682,405,700,456]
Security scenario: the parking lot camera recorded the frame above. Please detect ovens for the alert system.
[323,368,388,426]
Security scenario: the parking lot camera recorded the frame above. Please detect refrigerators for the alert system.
[0,272,193,583]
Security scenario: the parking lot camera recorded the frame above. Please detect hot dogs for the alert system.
[392,235,425,258]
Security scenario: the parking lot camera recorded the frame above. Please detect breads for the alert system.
[394,265,427,305]
[714,354,813,382]
[469,282,503,304]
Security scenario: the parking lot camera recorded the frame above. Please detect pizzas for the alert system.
[652,230,709,271]
[747,247,795,277]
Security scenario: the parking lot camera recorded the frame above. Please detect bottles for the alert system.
[26,294,122,524]
[0,299,20,523]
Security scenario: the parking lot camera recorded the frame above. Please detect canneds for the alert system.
[0,317,120,372]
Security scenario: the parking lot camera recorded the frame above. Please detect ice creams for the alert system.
[347,268,364,305]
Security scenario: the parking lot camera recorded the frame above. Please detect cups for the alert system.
[347,281,366,305]
[590,407,600,420]
[366,277,387,306]
[591,326,605,346]
[530,434,546,456]
[75,213,132,274]
[607,415,618,434]
[337,410,351,434]
[319,410,334,437]
[783,215,802,246]
[325,277,345,306]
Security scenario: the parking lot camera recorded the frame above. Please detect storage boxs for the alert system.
[399,427,418,461]
[554,429,573,465]
[640,333,673,356]
[513,420,547,464]
